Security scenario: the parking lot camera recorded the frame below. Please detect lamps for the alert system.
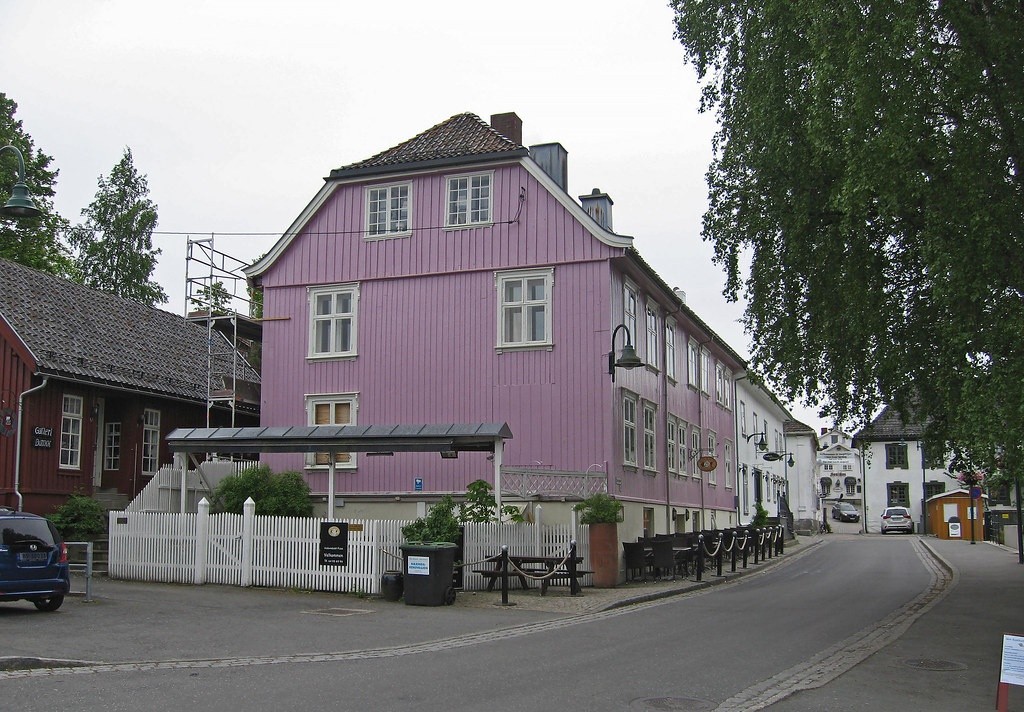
[609,324,645,383]
[763,473,785,487]
[137,413,145,427]
[739,464,747,474]
[366,452,393,456]
[90,404,99,422]
[779,452,795,467]
[748,432,768,451]
[440,451,458,458]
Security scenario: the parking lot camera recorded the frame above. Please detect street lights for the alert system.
[898,429,926,534]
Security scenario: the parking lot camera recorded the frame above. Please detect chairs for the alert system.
[888,510,892,515]
[622,527,766,584]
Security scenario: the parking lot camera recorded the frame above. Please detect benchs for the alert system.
[472,568,595,578]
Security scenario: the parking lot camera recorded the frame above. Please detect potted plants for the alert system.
[575,494,623,588]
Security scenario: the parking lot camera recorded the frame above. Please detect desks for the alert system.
[644,546,691,580]
[486,555,583,596]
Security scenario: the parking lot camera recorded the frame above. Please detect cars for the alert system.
[0,508,70,612]
[832,502,859,523]
[880,507,913,534]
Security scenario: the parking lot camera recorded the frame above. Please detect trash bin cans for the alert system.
[399,541,458,607]
